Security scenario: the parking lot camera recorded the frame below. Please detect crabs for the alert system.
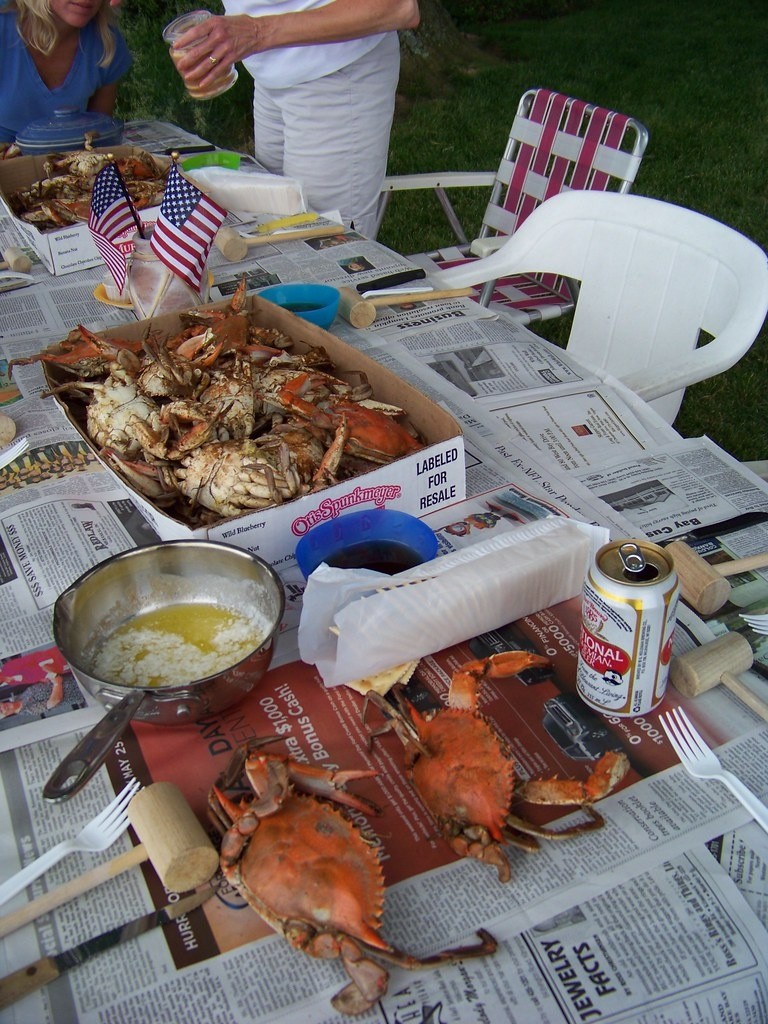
[205,650,631,1015]
[6,275,424,529]
[11,131,167,229]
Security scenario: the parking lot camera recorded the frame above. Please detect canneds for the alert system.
[576,539,682,717]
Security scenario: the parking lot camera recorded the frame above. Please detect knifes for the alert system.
[0,883,220,1010]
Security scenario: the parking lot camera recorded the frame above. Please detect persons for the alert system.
[110,0,420,240]
[0,0,130,143]
[311,235,348,249]
[0,646,84,718]
[349,259,374,270]
[400,302,417,310]
[0,441,96,490]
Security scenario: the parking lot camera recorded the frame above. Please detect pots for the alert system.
[41,538,285,805]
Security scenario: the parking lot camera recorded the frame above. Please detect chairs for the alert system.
[386,88,652,327]
[438,190,768,426]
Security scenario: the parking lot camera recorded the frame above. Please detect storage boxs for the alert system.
[42,295,468,574]
[0,145,213,276]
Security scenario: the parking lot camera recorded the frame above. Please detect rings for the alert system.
[208,55,218,66]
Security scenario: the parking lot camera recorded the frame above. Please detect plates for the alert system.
[93,284,135,309]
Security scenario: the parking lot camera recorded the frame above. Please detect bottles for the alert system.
[162,9,238,101]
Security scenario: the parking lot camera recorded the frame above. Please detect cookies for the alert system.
[329,576,455,698]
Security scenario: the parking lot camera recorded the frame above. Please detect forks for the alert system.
[1,777,146,906]
[238,229,311,238]
[659,705,768,835]
[0,271,34,280]
[0,437,30,470]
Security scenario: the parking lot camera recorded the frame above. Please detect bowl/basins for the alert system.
[182,152,240,172]
[257,283,340,332]
[102,270,128,301]
[294,509,441,576]
[15,106,124,156]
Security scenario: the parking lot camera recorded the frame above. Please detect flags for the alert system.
[150,160,227,293]
[88,165,145,296]
